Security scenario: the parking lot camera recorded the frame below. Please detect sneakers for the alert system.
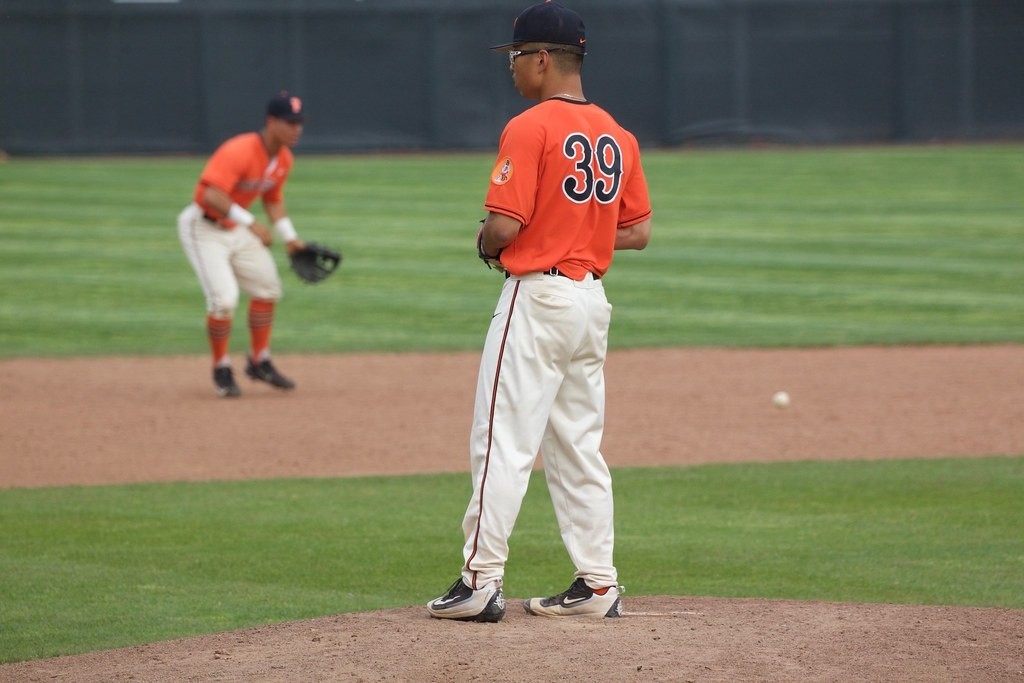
[426,577,507,621]
[524,576,625,618]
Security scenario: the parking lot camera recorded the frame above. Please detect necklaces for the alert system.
[549,92,587,103]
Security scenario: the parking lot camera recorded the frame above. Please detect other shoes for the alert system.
[245,357,294,389]
[210,366,240,398]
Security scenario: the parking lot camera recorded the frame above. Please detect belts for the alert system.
[505,265,600,280]
[204,213,218,224]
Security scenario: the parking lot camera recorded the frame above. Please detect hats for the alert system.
[489,0,586,51]
[267,95,305,121]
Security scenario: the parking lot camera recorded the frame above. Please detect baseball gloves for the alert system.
[288,241,343,283]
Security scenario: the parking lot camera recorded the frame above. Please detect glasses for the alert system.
[509,48,565,63]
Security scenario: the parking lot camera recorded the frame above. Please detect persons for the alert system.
[425,1,653,625]
[176,92,342,398]
